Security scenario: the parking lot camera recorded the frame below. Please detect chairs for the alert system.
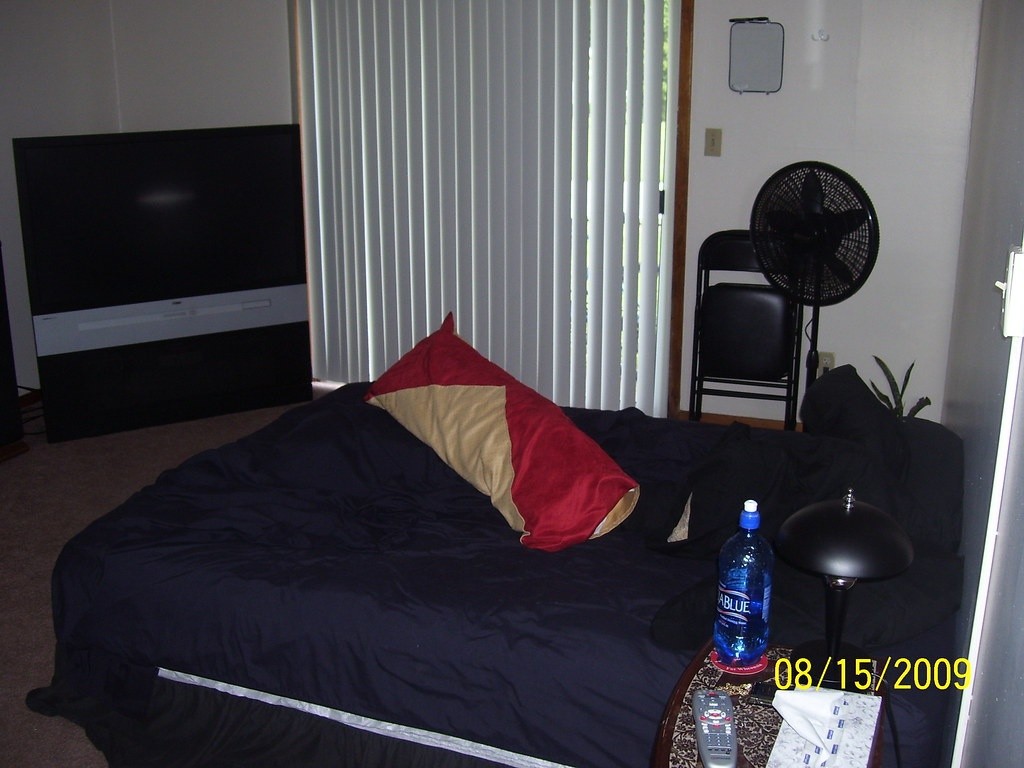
[688,230,806,431]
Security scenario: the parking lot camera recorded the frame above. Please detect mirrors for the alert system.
[728,17,785,95]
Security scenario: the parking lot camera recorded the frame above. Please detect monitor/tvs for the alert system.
[11,124,308,356]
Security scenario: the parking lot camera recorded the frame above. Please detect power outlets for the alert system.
[818,353,835,377]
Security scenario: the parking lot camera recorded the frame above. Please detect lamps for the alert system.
[777,486,916,691]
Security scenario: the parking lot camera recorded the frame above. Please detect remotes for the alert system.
[691,690,737,768]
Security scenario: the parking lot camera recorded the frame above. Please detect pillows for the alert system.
[364,311,642,552]
[646,364,965,649]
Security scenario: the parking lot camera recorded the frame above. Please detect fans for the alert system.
[749,161,880,431]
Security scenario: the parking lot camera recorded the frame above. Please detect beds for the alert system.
[25,384,965,768]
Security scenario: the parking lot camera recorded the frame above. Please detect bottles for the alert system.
[713,499,775,668]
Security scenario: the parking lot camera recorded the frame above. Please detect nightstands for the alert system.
[649,633,887,768]
